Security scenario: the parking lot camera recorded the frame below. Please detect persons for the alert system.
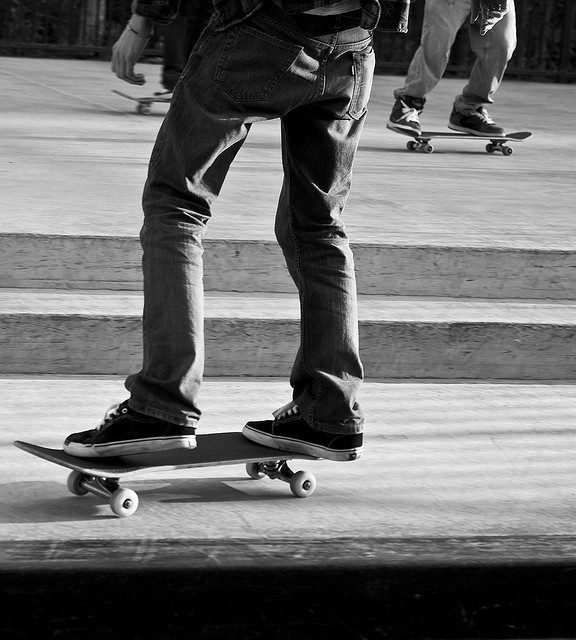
[387,0,517,137]
[160,0,214,90]
[64,0,382,462]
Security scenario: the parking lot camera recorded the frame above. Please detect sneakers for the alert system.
[243,402,363,462]
[387,97,422,137]
[63,397,198,457]
[447,106,506,137]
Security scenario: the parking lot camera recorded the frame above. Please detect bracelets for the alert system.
[128,20,155,38]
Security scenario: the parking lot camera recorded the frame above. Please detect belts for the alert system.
[291,8,363,36]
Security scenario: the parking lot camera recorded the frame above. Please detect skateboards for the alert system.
[112,89,173,114]
[14,431,322,518]
[385,126,532,156]
